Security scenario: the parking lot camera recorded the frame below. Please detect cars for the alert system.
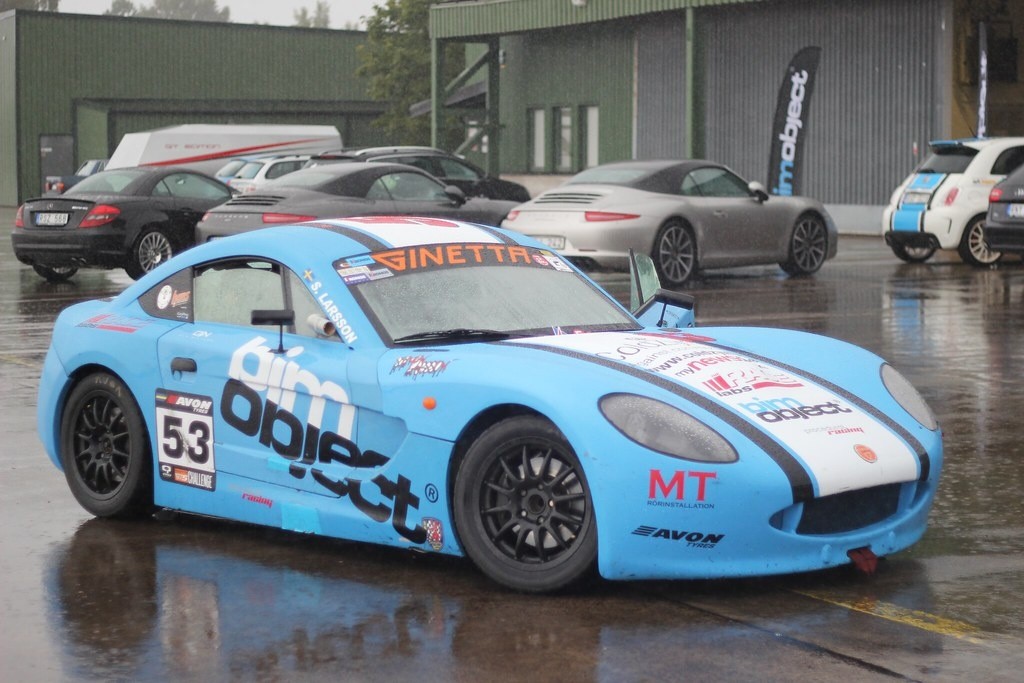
[11,167,241,284]
[502,159,838,290]
[36,216,944,596]
[43,518,946,682]
[880,137,1024,270]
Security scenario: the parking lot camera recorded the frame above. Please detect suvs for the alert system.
[46,123,533,246]
